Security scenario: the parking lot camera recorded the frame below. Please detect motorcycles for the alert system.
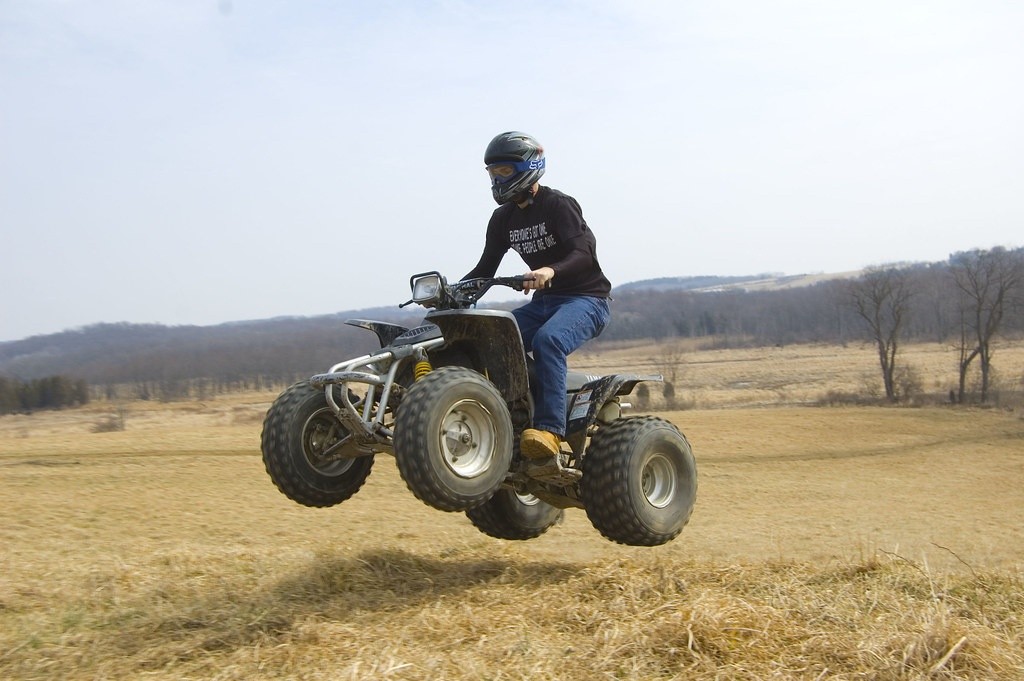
[261,272,699,547]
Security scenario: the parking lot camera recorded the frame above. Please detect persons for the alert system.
[413,131,612,460]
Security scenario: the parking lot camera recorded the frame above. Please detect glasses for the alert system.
[485,162,517,185]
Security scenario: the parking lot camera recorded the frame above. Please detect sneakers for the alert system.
[520,429,561,458]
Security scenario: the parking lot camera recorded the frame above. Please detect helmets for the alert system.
[485,131,545,205]
